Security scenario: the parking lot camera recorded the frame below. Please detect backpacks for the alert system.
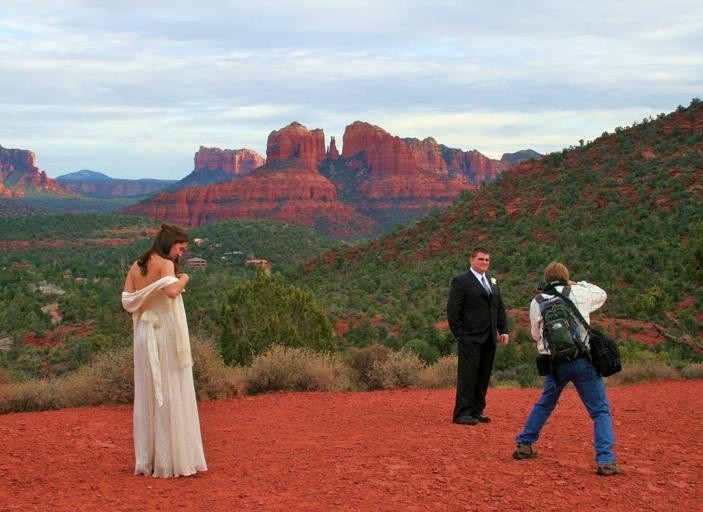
[534,285,588,364]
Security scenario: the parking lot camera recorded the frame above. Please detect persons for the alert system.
[121,223,208,480]
[444,247,512,427]
[508,261,624,476]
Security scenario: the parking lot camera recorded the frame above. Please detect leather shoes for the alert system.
[476,414,492,423]
[454,416,479,425]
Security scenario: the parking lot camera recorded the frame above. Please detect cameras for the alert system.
[536,280,546,292]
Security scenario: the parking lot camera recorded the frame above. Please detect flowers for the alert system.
[491,277,497,288]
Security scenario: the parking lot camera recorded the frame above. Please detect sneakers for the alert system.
[597,464,624,475]
[512,445,540,460]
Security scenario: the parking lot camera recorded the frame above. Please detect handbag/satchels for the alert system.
[588,330,622,379]
[536,355,551,376]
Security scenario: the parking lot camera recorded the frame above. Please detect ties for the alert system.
[481,277,492,298]
